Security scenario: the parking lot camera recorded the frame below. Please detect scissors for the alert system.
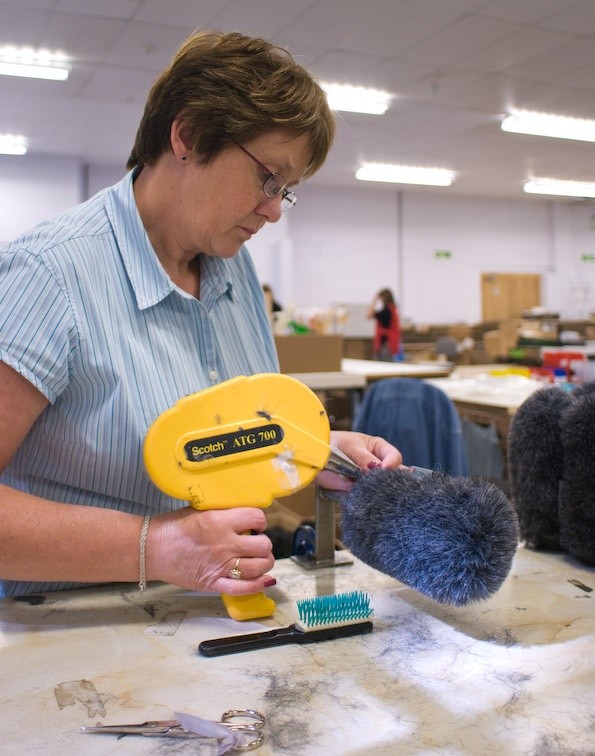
[84,709,266,751]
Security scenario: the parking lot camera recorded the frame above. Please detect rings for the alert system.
[228,558,240,578]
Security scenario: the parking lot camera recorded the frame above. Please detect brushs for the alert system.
[199,590,373,658]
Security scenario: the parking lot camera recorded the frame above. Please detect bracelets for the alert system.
[138,515,150,591]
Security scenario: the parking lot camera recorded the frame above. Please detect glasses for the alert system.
[238,143,298,211]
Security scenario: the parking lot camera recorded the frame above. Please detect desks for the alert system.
[422,363,548,493]
[0,537,595,756]
[282,372,367,431]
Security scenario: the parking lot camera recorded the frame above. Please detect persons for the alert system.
[367,289,404,362]
[262,286,281,312]
[1,26,410,598]
